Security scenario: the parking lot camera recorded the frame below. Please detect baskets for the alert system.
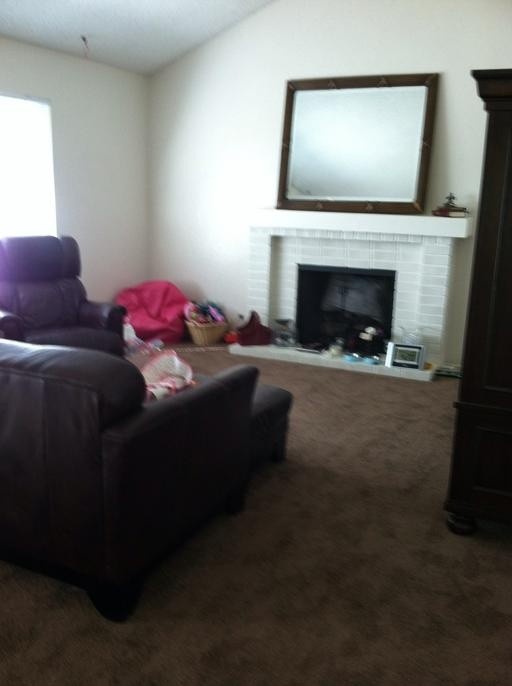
[183,317,230,347]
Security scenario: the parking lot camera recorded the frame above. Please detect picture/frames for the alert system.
[277,73,438,214]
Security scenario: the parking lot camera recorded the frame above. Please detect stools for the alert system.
[192,375,294,464]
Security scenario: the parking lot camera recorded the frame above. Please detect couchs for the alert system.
[0,236,127,357]
[0,338,292,621]
